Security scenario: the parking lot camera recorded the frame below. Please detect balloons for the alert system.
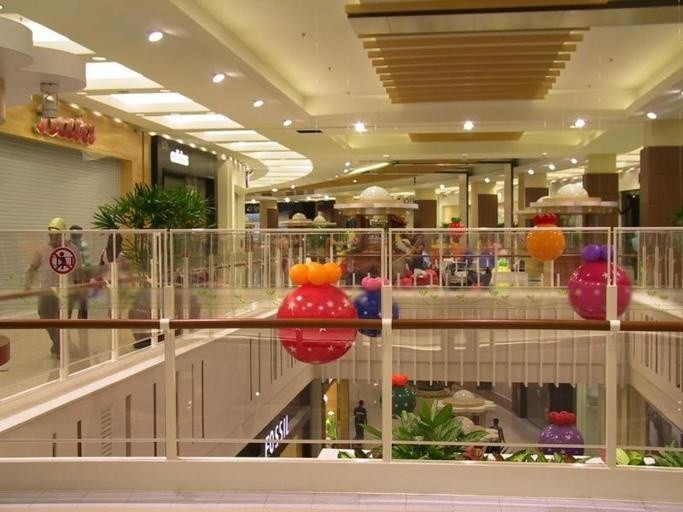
[379,374,416,416]
[276,262,398,364]
[527,213,632,321]
[540,410,583,456]
[448,216,466,238]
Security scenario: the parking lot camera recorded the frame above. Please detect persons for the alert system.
[489,418,508,443]
[67,225,92,318]
[354,400,368,440]
[326,411,337,448]
[24,218,89,359]
[100,233,130,319]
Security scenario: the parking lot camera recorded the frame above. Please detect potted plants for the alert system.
[91,182,217,339]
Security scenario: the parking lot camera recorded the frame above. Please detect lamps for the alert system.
[40,83,59,118]
